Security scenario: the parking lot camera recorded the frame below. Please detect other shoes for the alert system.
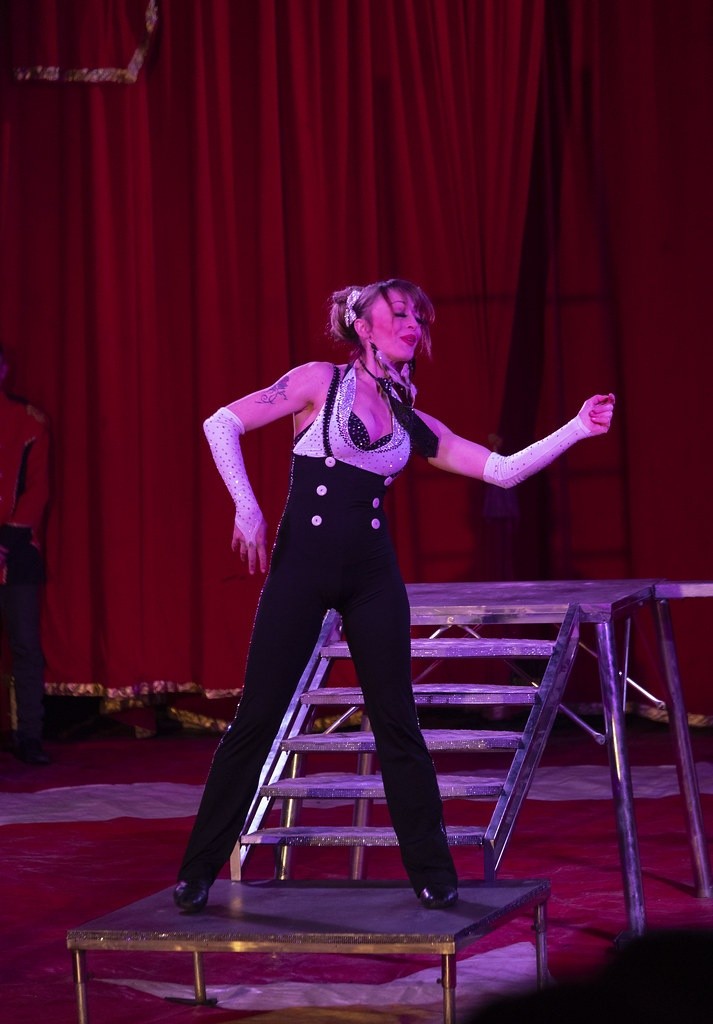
[19,736,52,768]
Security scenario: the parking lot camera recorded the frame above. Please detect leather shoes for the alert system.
[418,882,459,909]
[173,880,208,913]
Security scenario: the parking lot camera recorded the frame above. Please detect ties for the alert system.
[356,356,440,458]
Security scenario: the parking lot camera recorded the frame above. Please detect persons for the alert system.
[174,279,616,911]
[0,342,49,764]
